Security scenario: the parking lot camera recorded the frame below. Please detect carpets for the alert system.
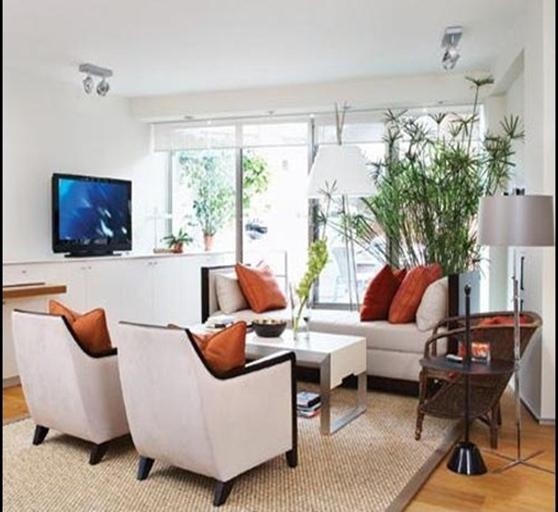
[2,378,477,512]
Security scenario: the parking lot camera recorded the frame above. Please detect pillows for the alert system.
[215,273,248,314]
[447,315,528,378]
[48,298,112,354]
[415,275,449,332]
[388,262,442,324]
[167,321,246,374]
[360,264,407,321]
[235,261,288,313]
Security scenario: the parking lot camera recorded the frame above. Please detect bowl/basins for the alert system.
[251,319,287,338]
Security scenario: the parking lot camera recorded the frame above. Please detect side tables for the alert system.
[419,284,519,476]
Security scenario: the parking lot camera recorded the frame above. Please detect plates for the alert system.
[225,322,253,333]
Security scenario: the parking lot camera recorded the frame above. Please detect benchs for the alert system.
[201,263,459,397]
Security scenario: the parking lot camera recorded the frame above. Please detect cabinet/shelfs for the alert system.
[1,250,224,389]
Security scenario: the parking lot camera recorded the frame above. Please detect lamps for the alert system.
[303,102,379,199]
[479,188,556,475]
[79,64,113,95]
[441,26,463,70]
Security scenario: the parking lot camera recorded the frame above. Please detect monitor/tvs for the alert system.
[51,173,133,258]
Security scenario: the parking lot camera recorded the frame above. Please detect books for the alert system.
[446,341,491,363]
[296,391,322,418]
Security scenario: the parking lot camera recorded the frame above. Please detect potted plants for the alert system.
[178,152,271,250]
[163,228,193,253]
[293,240,328,342]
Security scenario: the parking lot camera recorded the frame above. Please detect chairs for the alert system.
[10,308,131,465]
[116,321,297,506]
[414,311,542,448]
